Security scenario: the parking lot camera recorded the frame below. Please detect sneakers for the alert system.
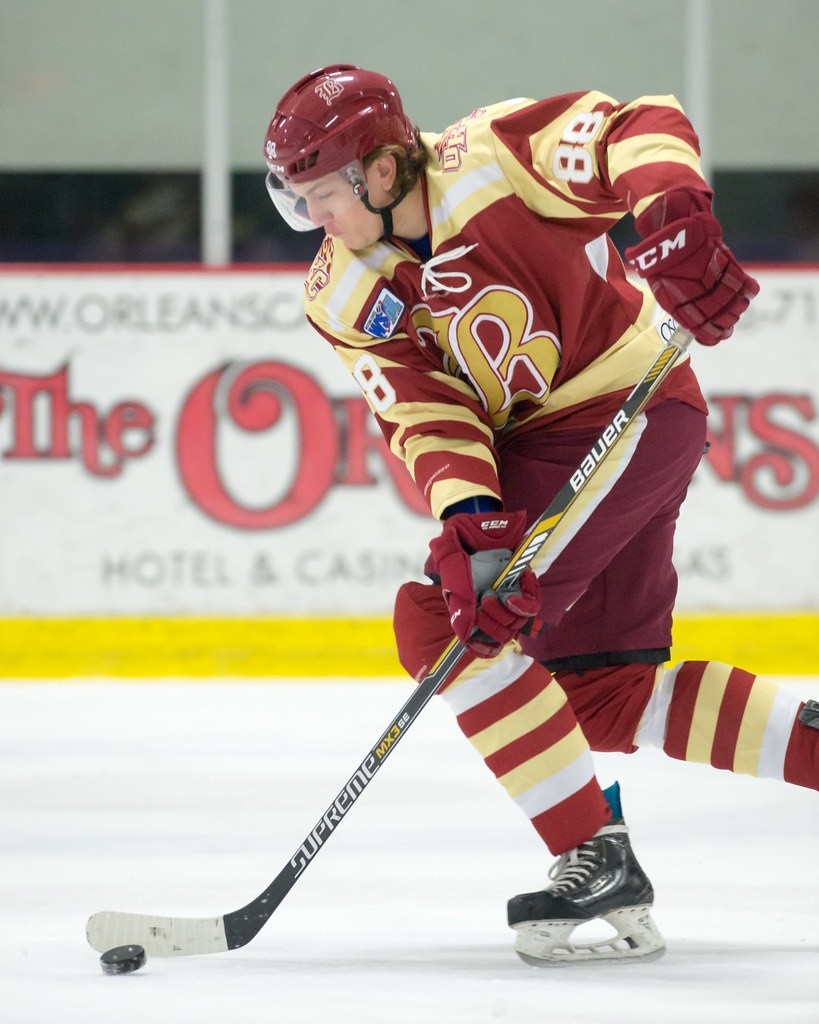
[507,780,666,962]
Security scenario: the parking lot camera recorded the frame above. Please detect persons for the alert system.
[262,65,816,965]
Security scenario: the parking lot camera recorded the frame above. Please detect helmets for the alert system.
[263,62,412,183]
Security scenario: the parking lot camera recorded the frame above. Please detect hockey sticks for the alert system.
[80,322,703,958]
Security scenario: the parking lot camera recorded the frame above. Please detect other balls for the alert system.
[99,944,146,976]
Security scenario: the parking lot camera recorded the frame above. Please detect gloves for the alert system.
[428,509,543,660]
[625,186,760,346]
